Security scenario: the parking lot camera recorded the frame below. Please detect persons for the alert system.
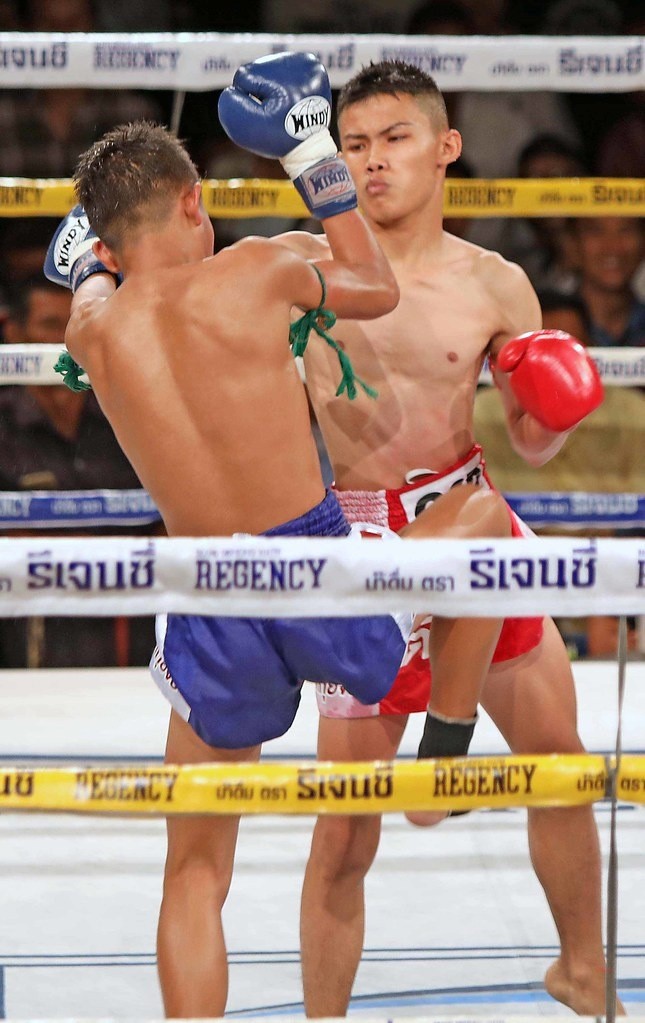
[0,0,645,666]
[44,49,513,1018]
[272,63,629,1017]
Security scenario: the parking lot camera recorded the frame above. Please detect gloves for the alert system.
[43,202,121,292]
[496,328,606,433]
[216,48,359,221]
